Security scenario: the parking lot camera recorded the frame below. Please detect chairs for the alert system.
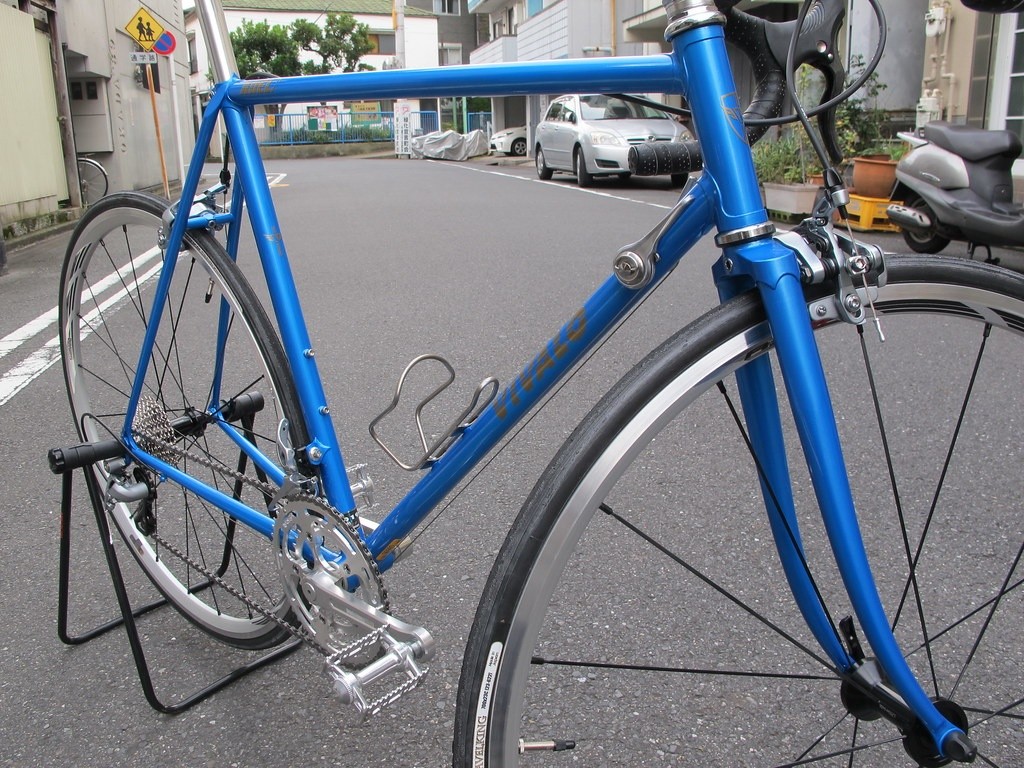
[611,107,629,118]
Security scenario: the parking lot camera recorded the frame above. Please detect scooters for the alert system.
[884,118,1024,266]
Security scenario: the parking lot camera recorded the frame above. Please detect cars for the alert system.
[534,92,695,189]
[489,125,527,157]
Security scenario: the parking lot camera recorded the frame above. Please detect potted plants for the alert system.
[749,53,912,215]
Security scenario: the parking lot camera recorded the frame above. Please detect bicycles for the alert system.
[46,0,1023,767]
[76,152,109,209]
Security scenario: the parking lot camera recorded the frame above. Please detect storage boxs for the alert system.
[834,193,903,232]
